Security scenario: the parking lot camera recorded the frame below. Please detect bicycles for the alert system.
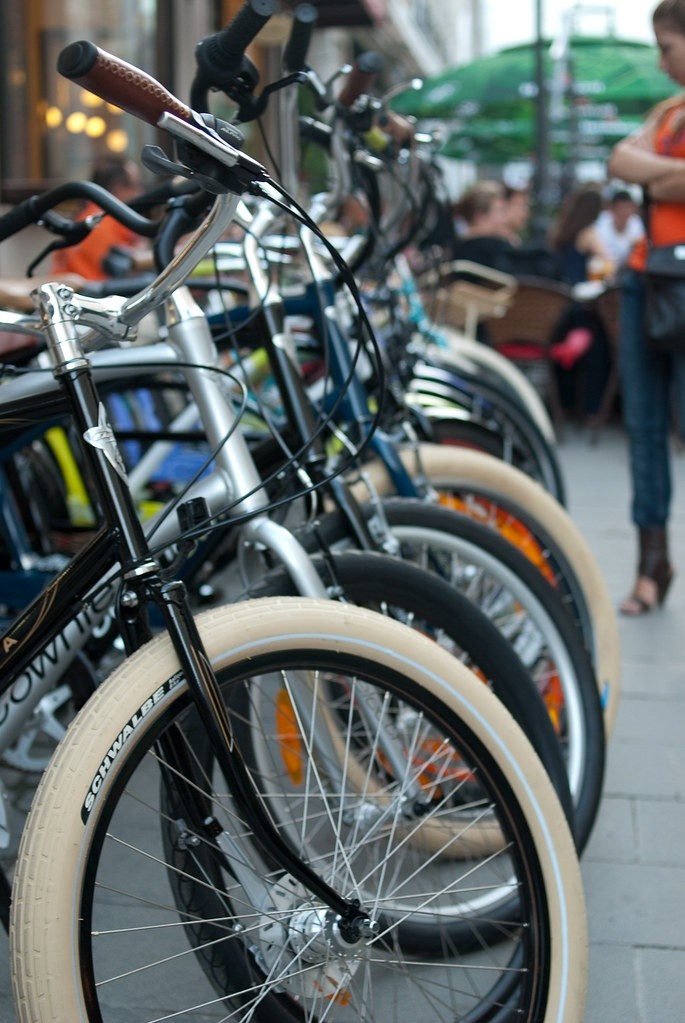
[1,0,617,1023]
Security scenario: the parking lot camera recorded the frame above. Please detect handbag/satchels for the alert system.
[640,242,685,347]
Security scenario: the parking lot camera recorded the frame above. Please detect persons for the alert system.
[606,1,685,618]
[50,146,645,421]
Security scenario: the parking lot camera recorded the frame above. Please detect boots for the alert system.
[619,525,673,614]
